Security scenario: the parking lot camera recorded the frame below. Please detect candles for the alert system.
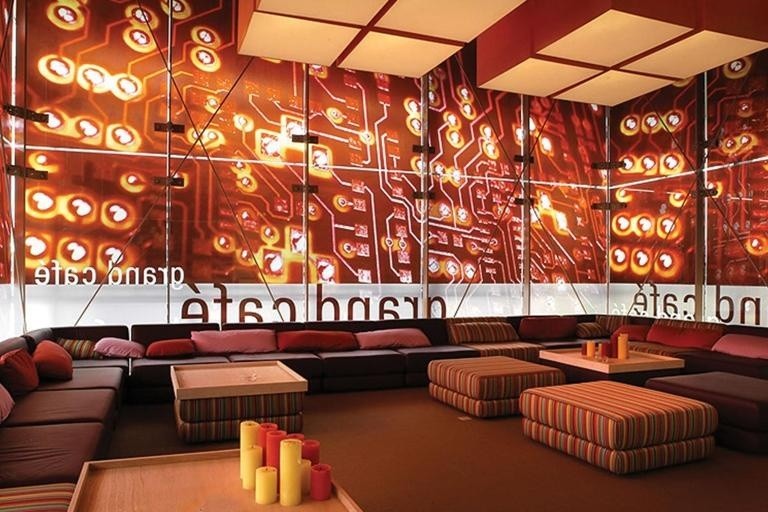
[238,418,333,507]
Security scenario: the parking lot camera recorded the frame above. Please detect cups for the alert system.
[241,369,259,383]
[593,351,611,364]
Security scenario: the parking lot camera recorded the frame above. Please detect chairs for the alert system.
[0,324,131,512]
